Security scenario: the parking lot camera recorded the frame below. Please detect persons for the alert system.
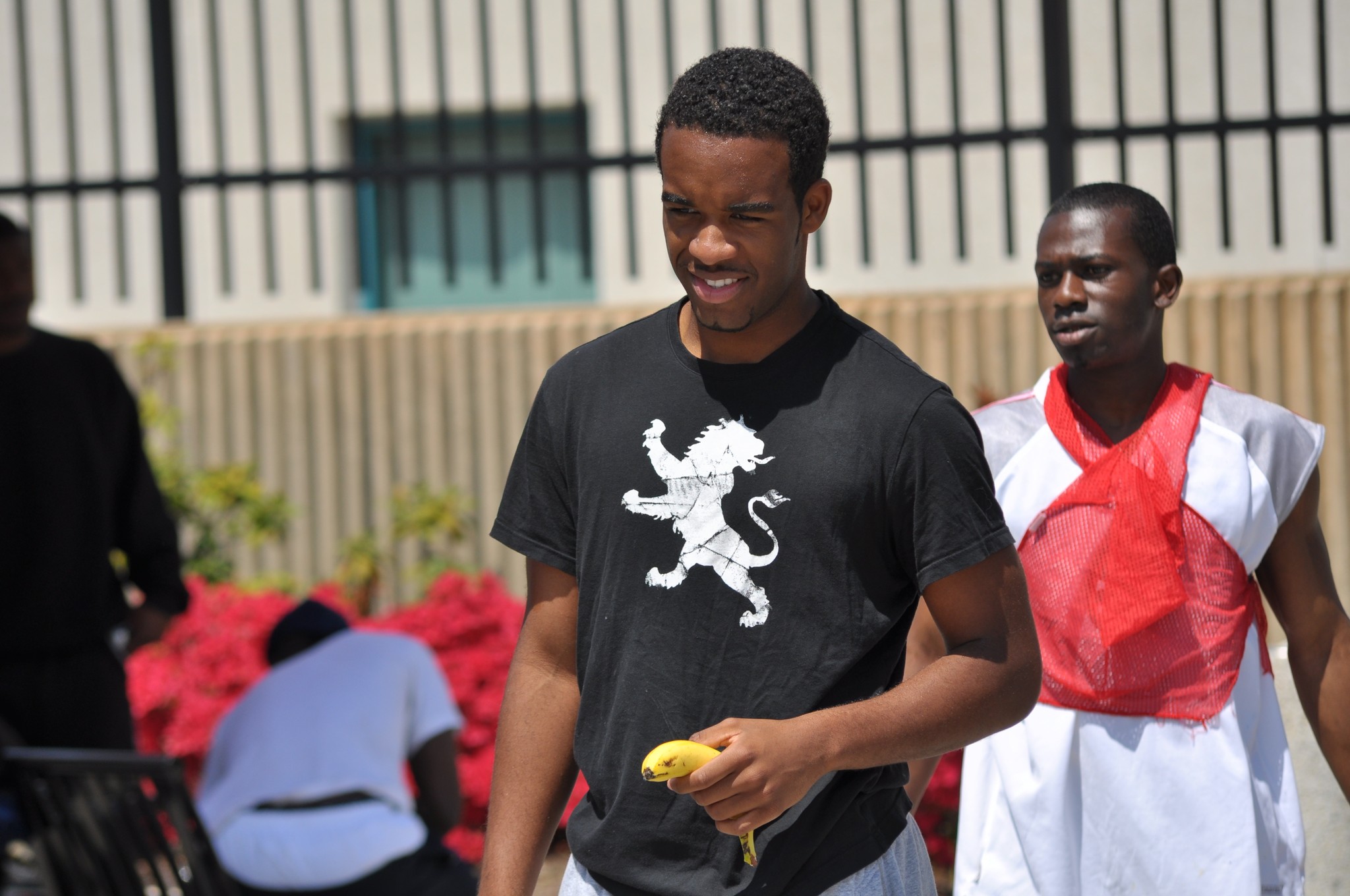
[896,181,1350,896]
[0,211,191,896]
[478,49,1041,896]
[192,599,479,896]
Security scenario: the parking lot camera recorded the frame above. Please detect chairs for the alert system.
[0,745,231,896]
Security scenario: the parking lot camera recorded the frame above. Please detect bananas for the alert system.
[641,740,757,866]
